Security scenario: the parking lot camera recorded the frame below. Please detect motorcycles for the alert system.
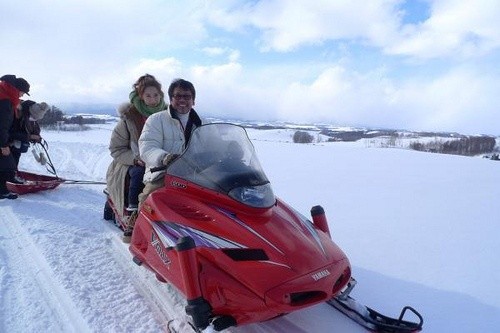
[101,121,427,332]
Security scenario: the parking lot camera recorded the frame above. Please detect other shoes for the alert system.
[0,191,18,199]
[8,177,24,184]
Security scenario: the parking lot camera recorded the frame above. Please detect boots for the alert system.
[122,208,137,243]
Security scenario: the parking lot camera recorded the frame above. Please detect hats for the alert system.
[137,74,161,100]
[29,101,49,120]
[14,78,31,96]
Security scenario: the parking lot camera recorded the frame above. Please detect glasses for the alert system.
[172,94,193,101]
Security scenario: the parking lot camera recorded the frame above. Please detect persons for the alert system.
[8,99,48,184]
[0,75,30,200]
[138,79,244,217]
[106,75,169,243]
